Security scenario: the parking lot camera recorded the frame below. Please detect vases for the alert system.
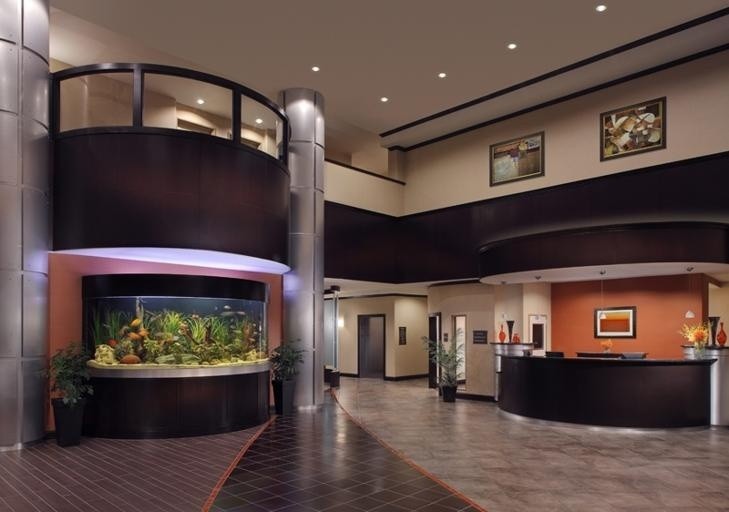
[693,347,706,361]
[717,322,727,347]
[499,324,505,343]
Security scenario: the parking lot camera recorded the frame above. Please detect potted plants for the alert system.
[31,340,95,448]
[267,337,309,416]
[420,328,468,403]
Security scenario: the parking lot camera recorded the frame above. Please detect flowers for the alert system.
[680,319,715,358]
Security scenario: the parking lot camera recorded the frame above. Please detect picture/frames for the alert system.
[599,96,667,161]
[594,306,637,338]
[489,130,549,186]
